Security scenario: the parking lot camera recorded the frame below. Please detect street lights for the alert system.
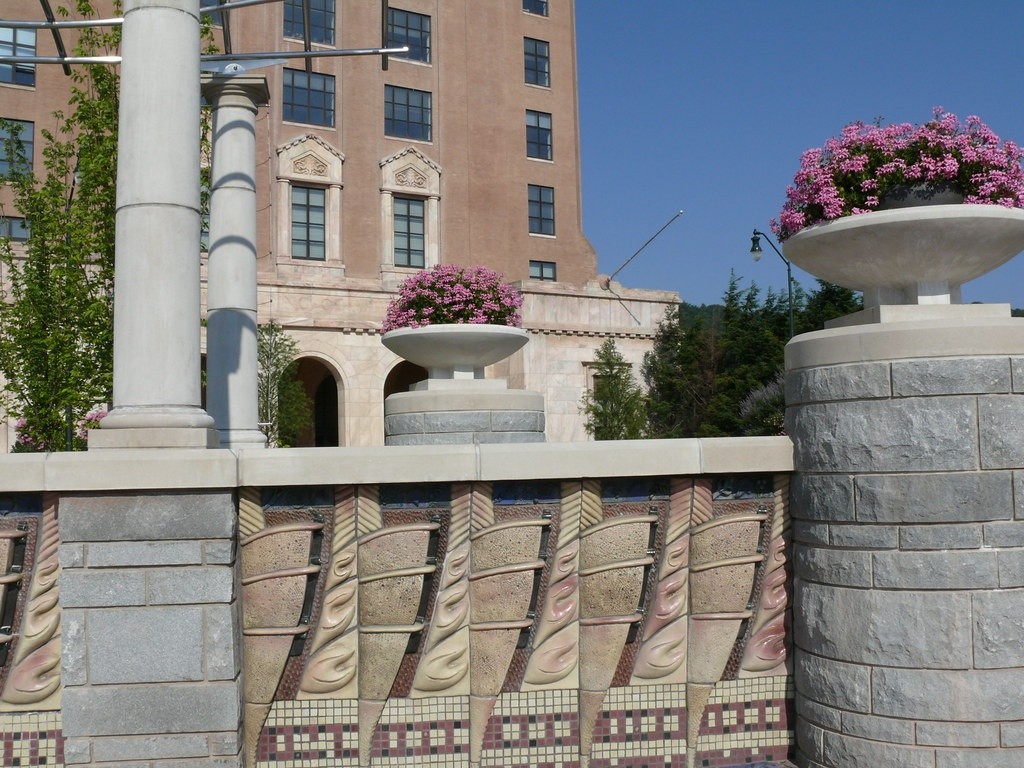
[749,229,794,337]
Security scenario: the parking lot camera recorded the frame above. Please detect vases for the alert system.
[381,324,529,379]
[781,184,1024,309]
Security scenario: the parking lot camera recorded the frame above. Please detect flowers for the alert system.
[379,264,522,334]
[771,104,1024,241]
